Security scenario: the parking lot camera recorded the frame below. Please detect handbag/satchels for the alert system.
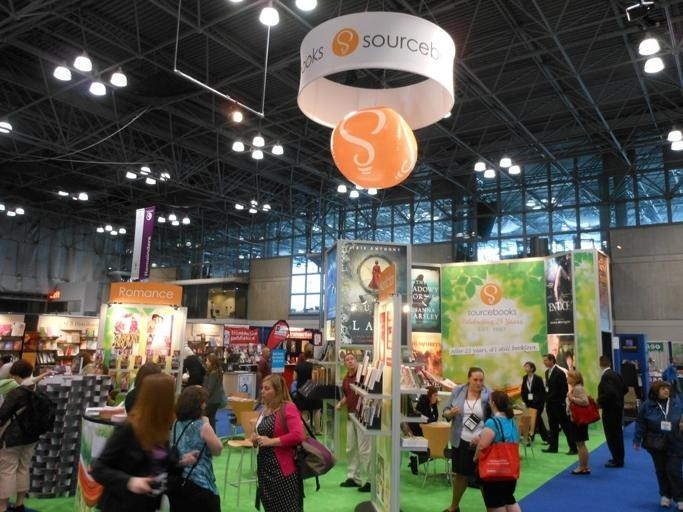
[478,416,520,481]
[569,389,599,428]
[279,400,336,479]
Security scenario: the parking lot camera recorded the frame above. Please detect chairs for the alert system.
[399,396,537,491]
[222,396,261,509]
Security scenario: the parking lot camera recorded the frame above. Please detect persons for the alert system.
[519,354,578,455]
[553,258,571,301]
[474,392,522,512]
[73,351,230,512]
[145,314,160,356]
[1,357,55,511]
[368,260,382,288]
[409,386,439,473]
[633,380,683,511]
[253,342,323,435]
[336,353,374,492]
[566,369,590,474]
[595,355,628,467]
[412,274,430,321]
[252,374,306,512]
[442,368,495,512]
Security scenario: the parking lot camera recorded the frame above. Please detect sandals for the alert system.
[571,467,591,474]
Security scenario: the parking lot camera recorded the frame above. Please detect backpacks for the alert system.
[9,384,56,436]
[166,419,205,505]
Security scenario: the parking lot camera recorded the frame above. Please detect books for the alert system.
[355,349,418,430]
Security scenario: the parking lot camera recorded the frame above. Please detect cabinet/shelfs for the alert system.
[348,383,392,437]
[0,315,99,373]
[400,387,430,452]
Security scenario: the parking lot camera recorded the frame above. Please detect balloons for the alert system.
[331,106,418,190]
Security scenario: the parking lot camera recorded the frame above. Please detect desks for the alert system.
[223,364,296,400]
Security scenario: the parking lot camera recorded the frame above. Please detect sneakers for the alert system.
[660,495,671,507]
[676,501,682,511]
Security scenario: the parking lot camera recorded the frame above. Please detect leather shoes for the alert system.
[340,478,361,487]
[606,462,624,467]
[569,448,577,454]
[358,482,370,491]
[542,447,558,452]
[8,503,25,511]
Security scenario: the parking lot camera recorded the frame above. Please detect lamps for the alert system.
[89,74,108,98]
[109,66,128,89]
[126,164,170,186]
[259,1,280,28]
[643,54,665,74]
[509,164,522,177]
[472,158,487,173]
[96,221,127,236]
[665,124,682,143]
[1,201,25,218]
[637,31,661,57]
[0,114,13,136]
[73,50,94,73]
[157,208,191,228]
[336,180,379,203]
[56,188,90,203]
[293,0,318,11]
[500,154,513,168]
[485,166,497,180]
[231,137,245,153]
[251,148,265,161]
[669,140,682,152]
[272,139,284,156]
[251,132,265,148]
[52,60,73,82]
[236,196,273,216]
[228,108,245,125]
